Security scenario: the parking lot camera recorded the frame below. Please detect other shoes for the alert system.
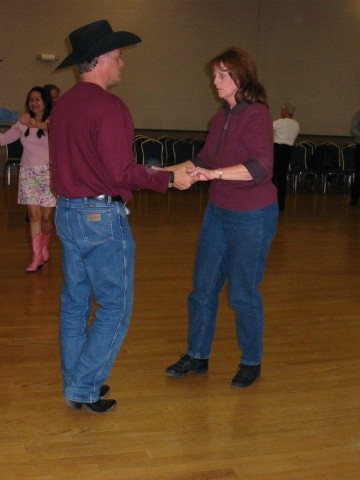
[67,385,112,410]
[165,354,209,375]
[25,213,30,224]
[230,363,261,388]
[75,398,119,417]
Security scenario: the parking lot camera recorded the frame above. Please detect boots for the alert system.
[26,233,44,272]
[42,229,52,261]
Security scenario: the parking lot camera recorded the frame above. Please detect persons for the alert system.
[349,111,360,205]
[151,47,279,389]
[0,84,61,272]
[47,19,200,414]
[273,101,300,211]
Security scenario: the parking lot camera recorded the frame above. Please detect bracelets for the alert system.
[169,171,173,189]
[218,168,223,179]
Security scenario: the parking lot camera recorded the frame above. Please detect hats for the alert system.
[54,19,142,70]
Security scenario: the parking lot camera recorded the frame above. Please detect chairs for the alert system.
[4,139,24,186]
[287,140,360,200]
[133,135,206,201]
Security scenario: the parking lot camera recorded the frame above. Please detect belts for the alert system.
[88,194,124,203]
